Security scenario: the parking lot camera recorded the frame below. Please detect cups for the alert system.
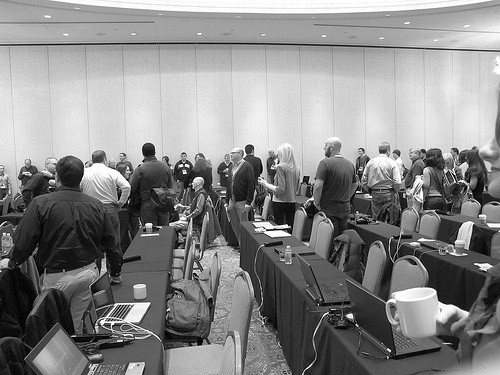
[145,222,153,234]
[454,240,466,255]
[364,194,370,198]
[386,287,439,339]
[479,214,487,227]
[133,284,147,300]
[279,249,285,262]
[439,246,448,256]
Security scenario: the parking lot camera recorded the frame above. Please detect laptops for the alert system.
[89,271,152,325]
[24,322,146,375]
[345,278,443,361]
[294,252,350,306]
[274,245,316,256]
[419,240,454,250]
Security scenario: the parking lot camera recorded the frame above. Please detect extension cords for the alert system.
[71,333,112,342]
[393,234,413,239]
[265,241,283,246]
[329,306,351,314]
[123,254,142,262]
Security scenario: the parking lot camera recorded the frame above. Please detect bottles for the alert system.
[6,233,13,255]
[1,233,7,254]
[285,246,292,264]
[355,211,360,224]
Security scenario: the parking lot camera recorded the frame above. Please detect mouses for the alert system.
[409,241,420,247]
[87,353,104,363]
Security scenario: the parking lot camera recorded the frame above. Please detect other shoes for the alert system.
[108,274,122,284]
[176,242,185,249]
[234,245,240,249]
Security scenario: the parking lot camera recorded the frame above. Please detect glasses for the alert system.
[230,152,240,156]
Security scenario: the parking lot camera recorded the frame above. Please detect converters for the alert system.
[98,339,124,349]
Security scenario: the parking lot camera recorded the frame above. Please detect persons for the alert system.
[0,155,123,335]
[392,57,499,375]
[1,136,487,240]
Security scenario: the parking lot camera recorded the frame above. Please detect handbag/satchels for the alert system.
[149,187,176,210]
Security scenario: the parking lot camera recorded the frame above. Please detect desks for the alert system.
[346,218,500,350]
[74,271,170,375]
[211,187,255,249]
[273,259,459,375]
[120,226,177,274]
[417,210,500,256]
[353,193,373,218]
[239,219,324,328]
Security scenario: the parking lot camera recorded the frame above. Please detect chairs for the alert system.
[0,178,500,375]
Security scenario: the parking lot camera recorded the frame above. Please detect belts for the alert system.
[371,188,391,191]
[45,259,96,273]
